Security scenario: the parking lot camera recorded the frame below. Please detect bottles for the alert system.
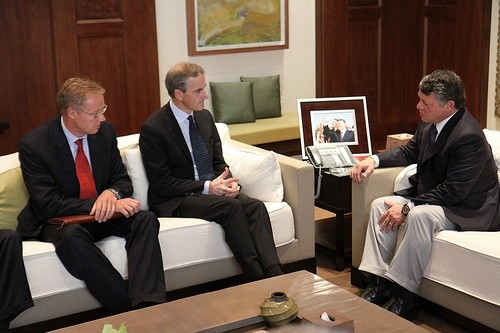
[258,291,299,326]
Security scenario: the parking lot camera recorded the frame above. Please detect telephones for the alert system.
[305,143,357,170]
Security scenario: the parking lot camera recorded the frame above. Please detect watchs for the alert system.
[109,188,119,198]
[402,203,410,216]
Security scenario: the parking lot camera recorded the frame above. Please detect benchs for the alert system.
[228,112,301,156]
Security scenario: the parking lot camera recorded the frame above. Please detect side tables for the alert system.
[289,149,382,271]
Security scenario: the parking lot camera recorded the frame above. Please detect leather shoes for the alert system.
[381,292,418,320]
[362,273,393,301]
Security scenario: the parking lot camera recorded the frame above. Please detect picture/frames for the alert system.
[185,0,289,57]
[296,96,372,160]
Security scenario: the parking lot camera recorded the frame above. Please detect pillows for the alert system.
[225,150,285,204]
[240,75,283,118]
[0,166,29,232]
[208,82,256,124]
[394,163,417,191]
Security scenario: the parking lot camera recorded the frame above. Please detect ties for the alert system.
[424,124,438,160]
[340,132,342,141]
[187,115,216,182]
[73,138,98,200]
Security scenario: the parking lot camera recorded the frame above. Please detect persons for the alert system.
[349,70,500,313]
[316,118,355,142]
[139,63,284,282]
[0,229,35,333]
[18,77,166,317]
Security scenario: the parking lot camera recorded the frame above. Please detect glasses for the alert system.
[70,104,108,119]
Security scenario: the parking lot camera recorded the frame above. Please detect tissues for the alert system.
[386,132,415,152]
[302,307,355,333]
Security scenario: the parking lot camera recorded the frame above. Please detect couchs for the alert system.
[0,124,316,328]
[352,129,500,333]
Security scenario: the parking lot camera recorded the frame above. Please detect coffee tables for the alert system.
[45,269,433,333]
